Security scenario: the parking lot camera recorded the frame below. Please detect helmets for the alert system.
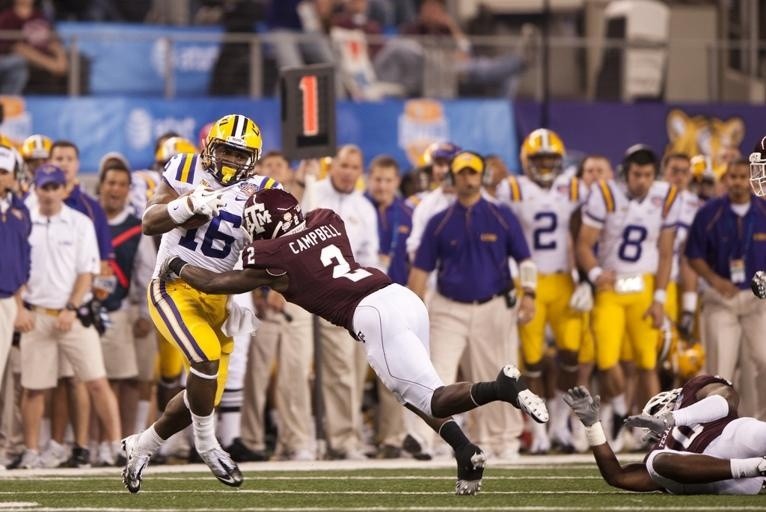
[200,114,262,186]
[154,136,198,161]
[520,128,568,187]
[749,136,766,196]
[240,188,307,244]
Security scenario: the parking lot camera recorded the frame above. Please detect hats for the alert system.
[433,143,483,173]
[0,134,65,187]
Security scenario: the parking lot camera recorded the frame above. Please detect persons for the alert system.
[1,0,766,497]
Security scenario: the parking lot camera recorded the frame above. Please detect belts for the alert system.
[23,301,63,316]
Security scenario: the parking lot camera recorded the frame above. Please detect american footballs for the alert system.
[159,189,212,226]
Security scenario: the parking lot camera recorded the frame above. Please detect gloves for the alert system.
[561,385,600,427]
[188,184,227,221]
[157,255,180,283]
[570,280,594,310]
[623,411,676,442]
[751,271,766,299]
[675,312,698,340]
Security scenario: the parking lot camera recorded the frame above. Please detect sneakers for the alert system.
[402,433,432,461]
[2,431,127,467]
[497,364,549,423]
[456,442,486,496]
[201,447,243,486]
[119,434,151,492]
